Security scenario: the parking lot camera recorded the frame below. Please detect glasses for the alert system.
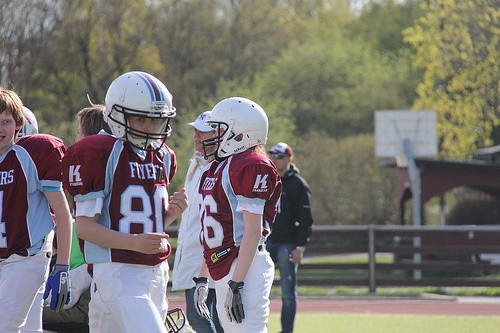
[269,152,290,159]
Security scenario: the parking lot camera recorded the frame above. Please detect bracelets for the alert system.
[296,247,305,252]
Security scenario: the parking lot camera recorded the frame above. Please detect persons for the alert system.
[267,143,313,333]
[0,88,72,333]
[193,97,282,333]
[16,94,121,333]
[61,72,189,333]
[172,111,224,333]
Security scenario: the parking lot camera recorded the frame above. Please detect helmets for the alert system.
[103,71,173,148]
[17,107,39,140]
[205,97,268,162]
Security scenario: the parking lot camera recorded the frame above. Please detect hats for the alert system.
[184,111,216,132]
[267,142,294,160]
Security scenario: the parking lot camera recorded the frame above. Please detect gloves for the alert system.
[43,264,72,312]
[223,279,245,323]
[192,276,211,320]
